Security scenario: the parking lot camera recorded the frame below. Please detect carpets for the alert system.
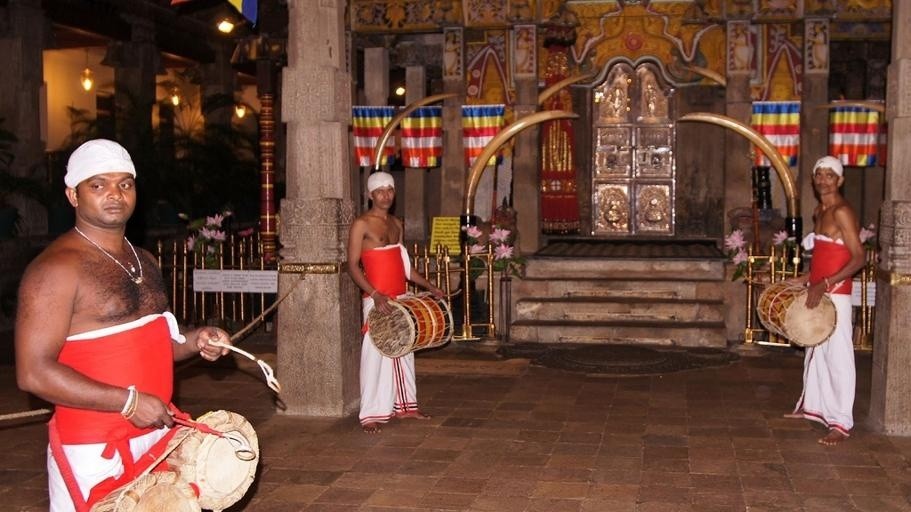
[498,339,741,376]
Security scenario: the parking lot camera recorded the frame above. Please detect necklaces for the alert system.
[74,225,145,285]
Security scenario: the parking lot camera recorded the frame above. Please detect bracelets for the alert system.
[122,385,140,419]
[823,277,830,291]
[370,289,377,298]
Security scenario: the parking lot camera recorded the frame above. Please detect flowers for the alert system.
[724,226,792,282]
[184,207,236,268]
[464,223,529,281]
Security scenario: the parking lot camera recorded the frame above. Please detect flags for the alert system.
[829,106,878,166]
[749,100,800,168]
[398,105,444,168]
[351,105,396,167]
[461,104,506,165]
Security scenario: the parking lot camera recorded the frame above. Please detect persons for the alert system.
[347,170,445,435]
[13,138,281,512]
[786,155,867,448]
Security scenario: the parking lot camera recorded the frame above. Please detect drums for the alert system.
[168,410,260,510]
[757,277,837,348]
[367,291,454,359]
[88,477,202,510]
